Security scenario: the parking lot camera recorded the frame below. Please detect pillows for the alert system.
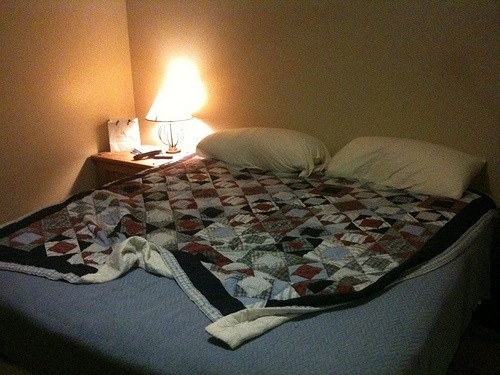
[326,135,486,201]
[195,127,331,179]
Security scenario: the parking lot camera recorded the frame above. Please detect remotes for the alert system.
[133,150,162,161]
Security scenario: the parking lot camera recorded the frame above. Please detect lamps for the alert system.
[146,94,192,154]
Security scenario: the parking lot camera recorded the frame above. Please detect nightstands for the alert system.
[92,148,189,188]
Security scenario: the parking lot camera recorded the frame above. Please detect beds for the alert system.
[0,143,498,375]
